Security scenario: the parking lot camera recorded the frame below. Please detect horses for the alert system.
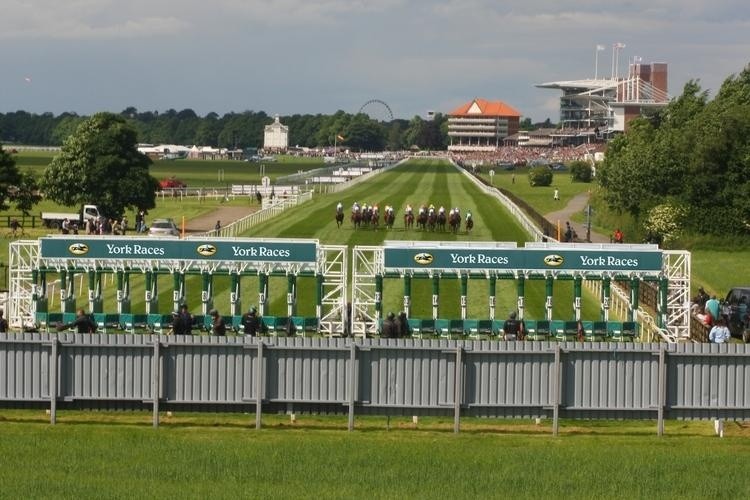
[351,207,379,232]
[404,212,413,229]
[384,209,394,231]
[335,206,345,229]
[465,213,473,233]
[416,212,461,232]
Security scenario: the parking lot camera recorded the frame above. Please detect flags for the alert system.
[612,42,626,48]
[628,55,643,61]
[596,44,604,51]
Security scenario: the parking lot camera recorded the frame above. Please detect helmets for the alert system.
[248,304,258,315]
[337,201,473,213]
[77,308,84,317]
[171,303,188,317]
[209,309,218,315]
[387,311,407,321]
[509,311,517,319]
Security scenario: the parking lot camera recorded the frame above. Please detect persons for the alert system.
[511,172,516,184]
[0,308,9,332]
[56,307,96,333]
[214,220,223,236]
[382,311,410,338]
[171,303,191,335]
[209,309,226,336]
[502,311,520,340]
[541,226,550,242]
[61,217,79,235]
[692,286,732,343]
[613,228,623,243]
[738,296,748,321]
[335,202,345,212]
[352,202,394,215]
[255,190,288,204]
[136,209,148,234]
[85,217,128,235]
[553,187,560,201]
[404,203,472,219]
[241,306,260,337]
[564,221,578,241]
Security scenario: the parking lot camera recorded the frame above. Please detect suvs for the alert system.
[149,217,180,237]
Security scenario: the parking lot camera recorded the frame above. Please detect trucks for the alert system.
[39,203,107,230]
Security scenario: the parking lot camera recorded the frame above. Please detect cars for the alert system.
[715,286,750,343]
